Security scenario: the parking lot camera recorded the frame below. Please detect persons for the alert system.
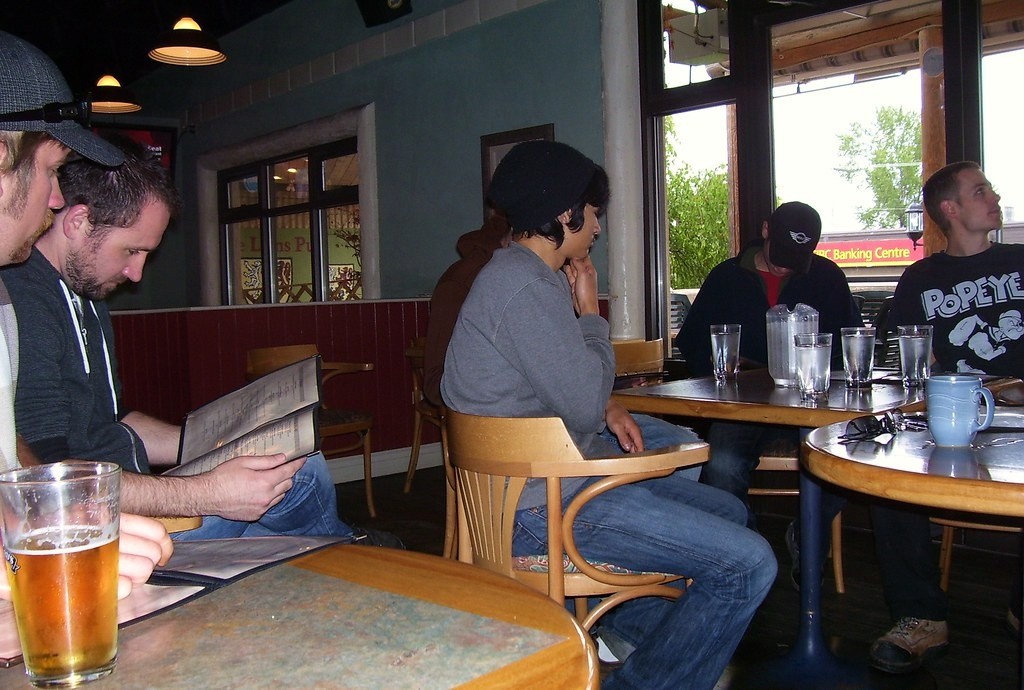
[440,138,777,690]
[869,162,1024,671]
[0,129,406,551]
[424,209,512,406]
[0,34,173,601]
[679,202,866,592]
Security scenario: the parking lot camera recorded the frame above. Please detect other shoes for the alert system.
[787,522,826,592]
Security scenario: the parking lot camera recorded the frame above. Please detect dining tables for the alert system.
[0,543,600,690]
[614,367,1024,690]
[801,406,1023,516]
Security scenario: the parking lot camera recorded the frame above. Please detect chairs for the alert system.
[747,437,845,593]
[443,410,710,631]
[613,338,663,376]
[403,337,443,493]
[670,290,904,363]
[245,343,377,518]
[929,516,1021,591]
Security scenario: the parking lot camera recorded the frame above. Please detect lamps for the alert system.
[149,15,227,66]
[905,195,925,250]
[88,74,144,114]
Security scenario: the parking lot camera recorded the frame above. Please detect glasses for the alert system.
[839,417,895,443]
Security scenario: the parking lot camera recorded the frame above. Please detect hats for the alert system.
[0,29,126,166]
[768,201,821,275]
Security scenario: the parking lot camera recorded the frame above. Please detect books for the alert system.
[161,354,322,480]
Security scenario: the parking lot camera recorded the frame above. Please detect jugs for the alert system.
[766,303,819,389]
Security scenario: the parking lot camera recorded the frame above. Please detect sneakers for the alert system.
[869,614,949,673]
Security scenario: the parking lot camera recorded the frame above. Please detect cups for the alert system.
[0,462,121,689]
[710,324,740,383]
[840,327,876,389]
[898,326,933,387]
[925,376,995,448]
[795,333,833,401]
